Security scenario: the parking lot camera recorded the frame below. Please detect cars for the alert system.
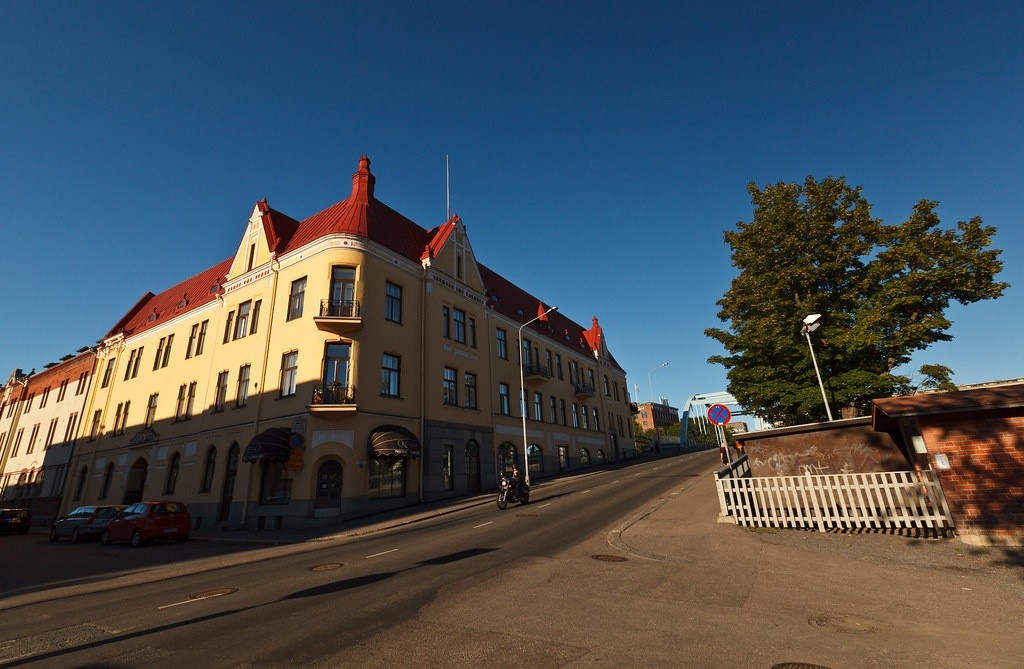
[100,499,192,547]
[0,507,32,536]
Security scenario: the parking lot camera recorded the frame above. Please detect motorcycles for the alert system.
[496,471,529,511]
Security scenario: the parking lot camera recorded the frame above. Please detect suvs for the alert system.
[50,505,121,543]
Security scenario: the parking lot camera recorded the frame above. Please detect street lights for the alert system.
[519,307,558,486]
[649,362,667,455]
[803,314,834,421]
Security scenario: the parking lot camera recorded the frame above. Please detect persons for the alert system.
[719,441,733,465]
[512,464,522,498]
[734,440,746,458]
